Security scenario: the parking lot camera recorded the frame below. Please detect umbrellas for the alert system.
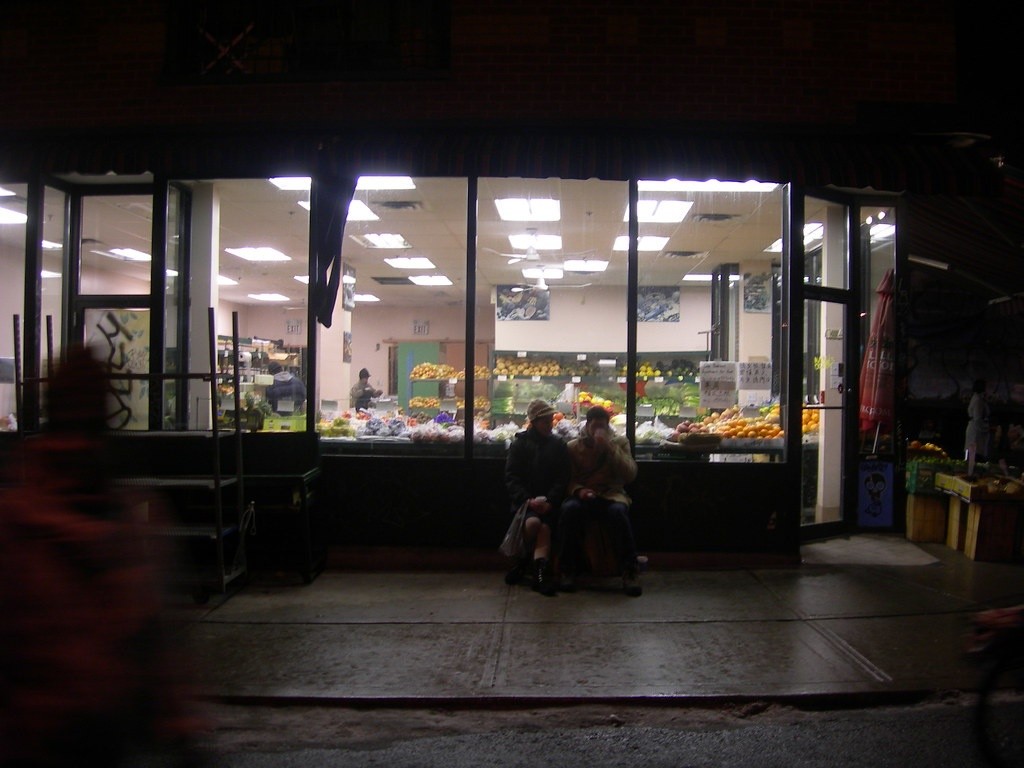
[858,267,897,453]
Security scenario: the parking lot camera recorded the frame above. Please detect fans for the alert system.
[511,270,591,293]
[484,228,596,265]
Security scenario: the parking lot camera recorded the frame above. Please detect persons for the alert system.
[505,400,599,592]
[965,379,997,463]
[350,368,383,413]
[559,406,640,597]
[265,361,306,417]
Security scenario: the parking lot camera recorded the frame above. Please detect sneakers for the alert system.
[623,562,642,596]
[559,569,578,592]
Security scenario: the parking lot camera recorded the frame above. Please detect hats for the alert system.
[359,369,371,378]
[268,362,282,374]
[527,401,558,422]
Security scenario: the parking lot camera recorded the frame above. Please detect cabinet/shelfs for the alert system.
[13,307,326,595]
[408,344,709,426]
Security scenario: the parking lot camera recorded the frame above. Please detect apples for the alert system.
[675,406,738,433]
[493,354,560,376]
[410,362,490,408]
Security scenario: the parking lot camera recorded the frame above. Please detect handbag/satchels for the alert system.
[499,498,530,556]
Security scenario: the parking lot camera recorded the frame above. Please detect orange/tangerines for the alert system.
[802,409,819,433]
[925,442,948,458]
[714,417,785,439]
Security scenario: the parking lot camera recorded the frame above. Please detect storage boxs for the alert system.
[904,460,1024,561]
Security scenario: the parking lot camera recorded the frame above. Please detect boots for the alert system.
[532,557,556,596]
[505,549,529,584]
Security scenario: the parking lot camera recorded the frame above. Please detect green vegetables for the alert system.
[633,383,711,422]
